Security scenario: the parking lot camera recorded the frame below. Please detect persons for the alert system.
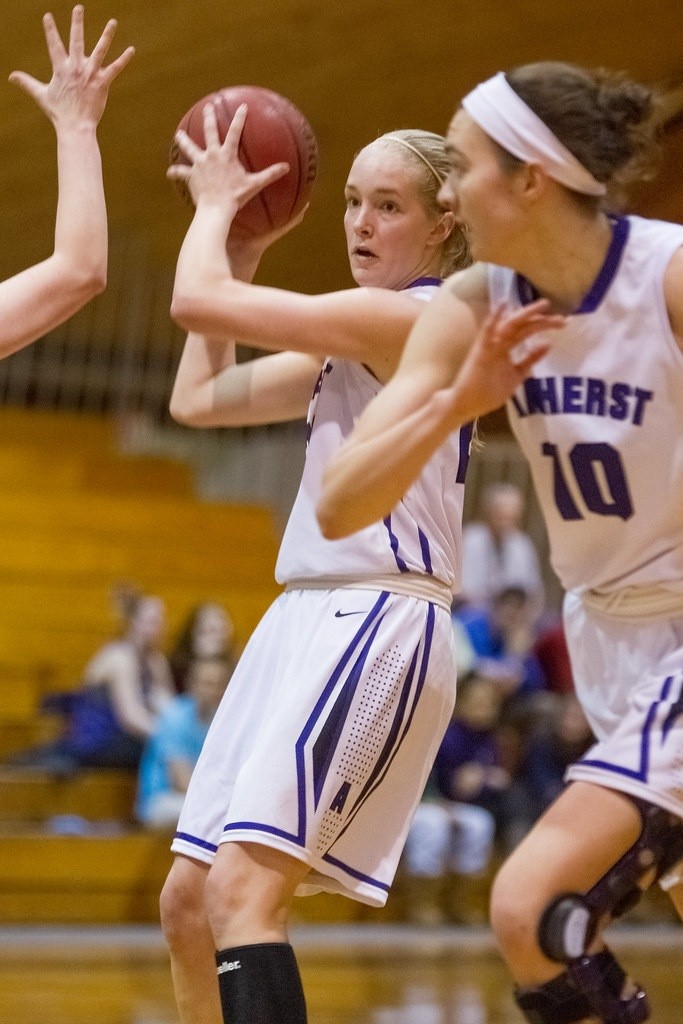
[406,586,597,928]
[173,603,228,693]
[133,659,226,828]
[65,594,173,771]
[317,59,683,1024]
[0,3,136,361]
[460,479,544,603]
[158,100,476,1024]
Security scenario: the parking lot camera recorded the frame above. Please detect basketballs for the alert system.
[170,85,318,236]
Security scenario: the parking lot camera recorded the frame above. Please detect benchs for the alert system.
[0,406,508,926]
